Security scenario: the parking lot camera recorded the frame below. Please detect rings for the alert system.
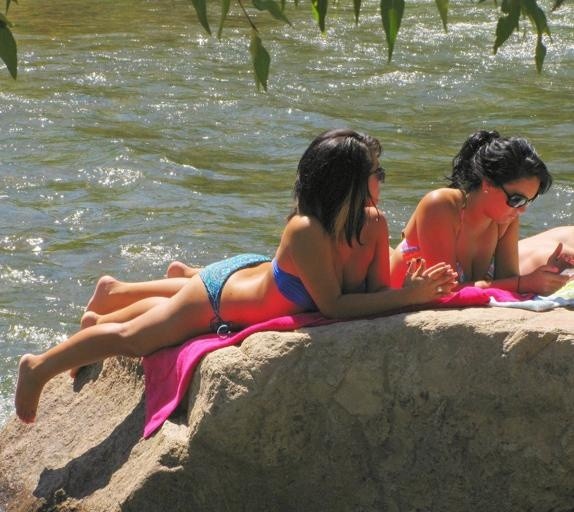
[436,286,442,292]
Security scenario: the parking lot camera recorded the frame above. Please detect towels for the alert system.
[143,287,534,437]
[486,280,574,312]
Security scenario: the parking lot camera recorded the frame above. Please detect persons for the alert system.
[79,129,574,322]
[14,128,461,425]
[516,224,574,275]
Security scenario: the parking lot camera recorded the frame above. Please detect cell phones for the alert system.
[560,267,574,277]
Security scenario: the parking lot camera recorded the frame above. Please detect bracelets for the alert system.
[516,276,522,292]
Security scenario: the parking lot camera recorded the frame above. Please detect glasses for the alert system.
[498,184,538,209]
[369,165,386,184]
